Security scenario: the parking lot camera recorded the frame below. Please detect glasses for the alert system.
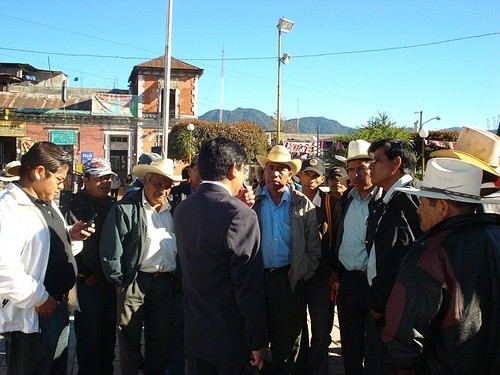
[242,166,248,175]
[48,170,67,185]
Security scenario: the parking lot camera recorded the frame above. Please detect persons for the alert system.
[167,153,202,215]
[249,143,321,375]
[124,173,134,194]
[379,156,500,375]
[324,139,400,375]
[172,135,271,375]
[362,136,424,375]
[109,172,121,200]
[99,158,180,375]
[322,167,358,375]
[294,157,346,375]
[121,153,164,199]
[0,140,97,375]
[64,157,118,375]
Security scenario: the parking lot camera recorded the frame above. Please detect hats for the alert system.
[394,158,495,204]
[335,139,374,163]
[430,126,500,179]
[0,160,22,182]
[138,153,161,163]
[83,157,118,178]
[133,158,188,182]
[299,158,325,176]
[256,145,302,177]
[329,167,347,178]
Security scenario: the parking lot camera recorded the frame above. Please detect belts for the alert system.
[266,266,290,276]
[78,272,90,278]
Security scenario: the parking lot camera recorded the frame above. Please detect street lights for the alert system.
[418,110,441,177]
[276,17,294,145]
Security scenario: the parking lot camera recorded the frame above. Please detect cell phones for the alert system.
[84,214,98,230]
